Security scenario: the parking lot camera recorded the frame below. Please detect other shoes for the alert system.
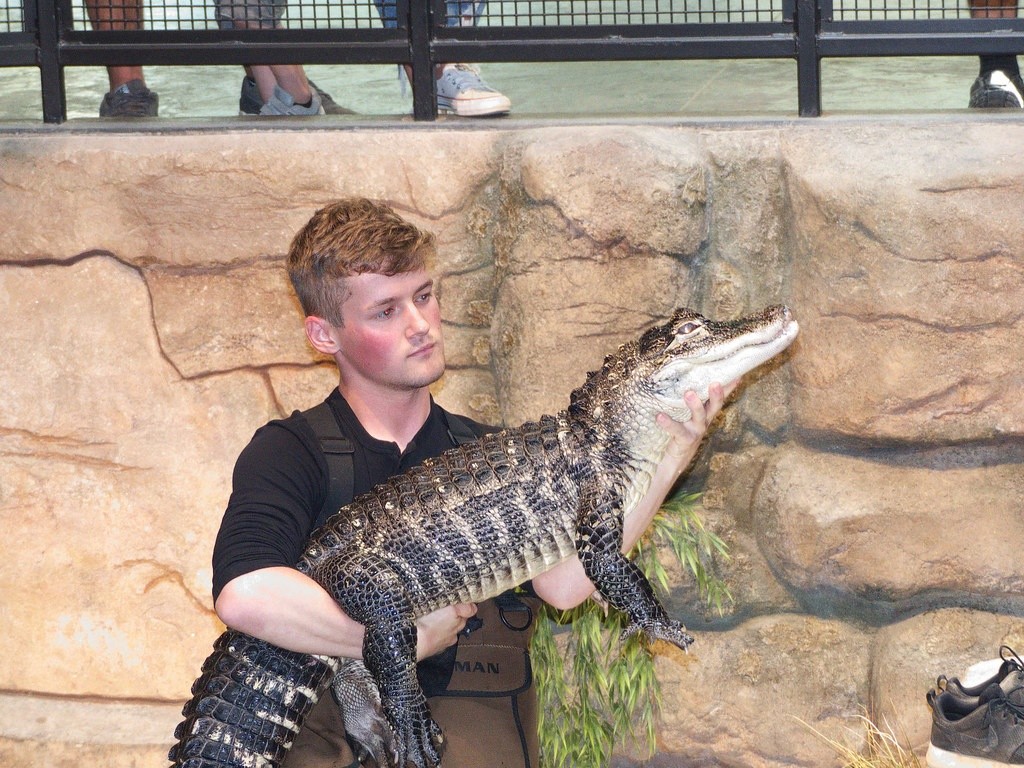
[968,69,1024,108]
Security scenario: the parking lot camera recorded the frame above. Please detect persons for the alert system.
[968,0,1024,110]
[83,1,160,118]
[213,0,515,115]
[212,201,747,767]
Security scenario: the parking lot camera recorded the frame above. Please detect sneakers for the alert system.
[260,83,329,115]
[926,645,1024,768]
[99,79,158,118]
[239,75,363,116]
[437,63,511,116]
